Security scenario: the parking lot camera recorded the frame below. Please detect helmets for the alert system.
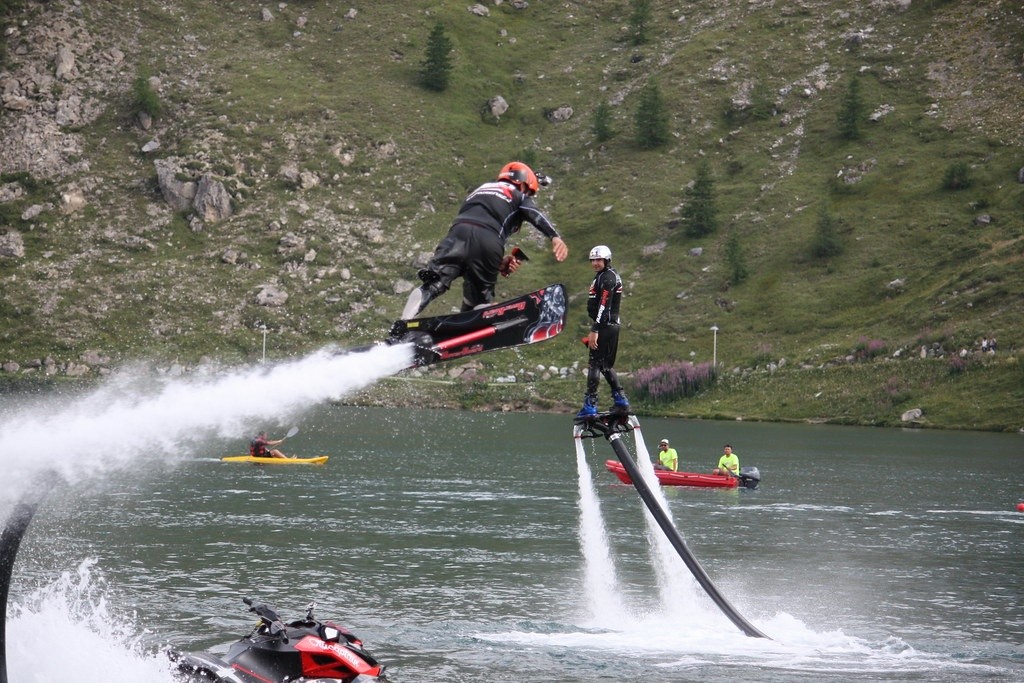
[589,245,612,260]
[498,162,538,195]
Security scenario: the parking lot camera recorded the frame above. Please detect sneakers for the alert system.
[608,390,629,411]
[577,396,598,418]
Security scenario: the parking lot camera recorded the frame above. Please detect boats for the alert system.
[222,454,329,466]
[606,457,761,490]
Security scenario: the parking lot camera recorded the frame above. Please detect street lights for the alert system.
[710,326,719,367]
[259,325,266,371]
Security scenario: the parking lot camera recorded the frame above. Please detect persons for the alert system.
[577,244,632,415]
[713,443,739,477]
[401,161,568,322]
[982,334,998,359]
[654,439,679,473]
[250,429,298,459]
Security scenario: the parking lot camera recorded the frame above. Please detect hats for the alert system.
[661,439,669,444]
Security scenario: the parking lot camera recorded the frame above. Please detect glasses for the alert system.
[661,445,667,446]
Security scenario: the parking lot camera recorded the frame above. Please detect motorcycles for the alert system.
[164,595,392,682]
